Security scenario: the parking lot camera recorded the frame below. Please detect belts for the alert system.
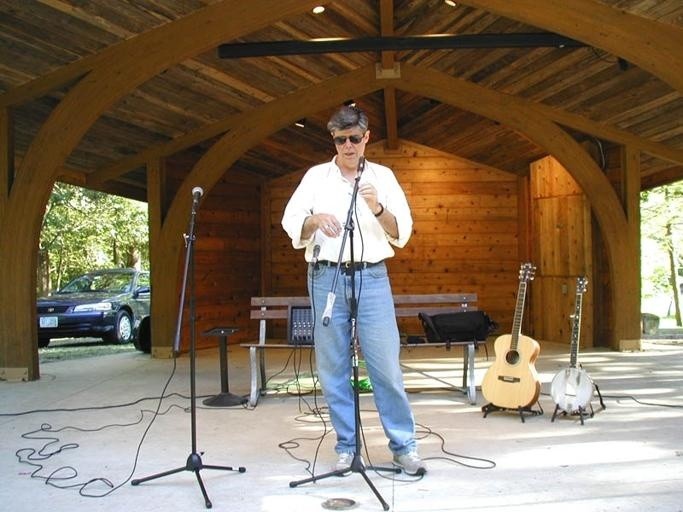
[318,260,383,271]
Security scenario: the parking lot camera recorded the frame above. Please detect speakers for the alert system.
[288,303,316,345]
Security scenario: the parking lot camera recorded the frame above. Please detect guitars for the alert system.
[482,263,541,410]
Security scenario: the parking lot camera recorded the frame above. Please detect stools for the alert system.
[200,325,248,407]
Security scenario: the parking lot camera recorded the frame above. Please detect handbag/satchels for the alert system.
[418,311,491,342]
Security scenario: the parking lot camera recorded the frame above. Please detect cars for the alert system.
[36,268,150,348]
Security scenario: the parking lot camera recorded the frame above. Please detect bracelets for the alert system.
[374,202,384,218]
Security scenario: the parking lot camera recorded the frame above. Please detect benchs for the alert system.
[241,292,480,410]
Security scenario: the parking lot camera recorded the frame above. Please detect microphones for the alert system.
[191,186,204,205]
[355,155,365,182]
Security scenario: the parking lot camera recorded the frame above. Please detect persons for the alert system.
[279,104,428,476]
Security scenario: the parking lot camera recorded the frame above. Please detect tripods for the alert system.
[288,194,401,512]
[131,221,246,509]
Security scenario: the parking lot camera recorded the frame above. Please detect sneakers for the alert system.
[336,453,366,476]
[392,451,427,475]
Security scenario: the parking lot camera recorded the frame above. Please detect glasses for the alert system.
[332,133,364,144]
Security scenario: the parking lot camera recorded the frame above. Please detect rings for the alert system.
[323,224,330,230]
